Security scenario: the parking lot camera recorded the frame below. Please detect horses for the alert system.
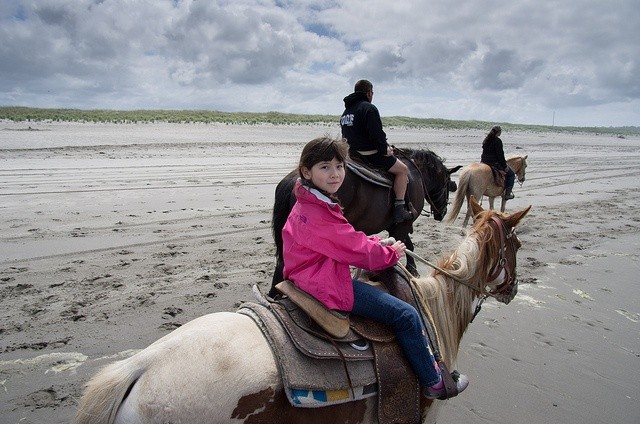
[446,154,529,236]
[69,193,534,424]
[267,146,464,299]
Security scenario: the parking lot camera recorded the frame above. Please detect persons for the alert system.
[339,80,413,224]
[481,126,515,200]
[281,136,470,401]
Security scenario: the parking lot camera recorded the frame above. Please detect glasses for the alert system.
[370,91,373,94]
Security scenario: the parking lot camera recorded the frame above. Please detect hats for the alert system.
[492,126,502,132]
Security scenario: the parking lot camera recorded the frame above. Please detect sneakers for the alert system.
[505,194,513,200]
[423,373,469,400]
[395,205,413,225]
